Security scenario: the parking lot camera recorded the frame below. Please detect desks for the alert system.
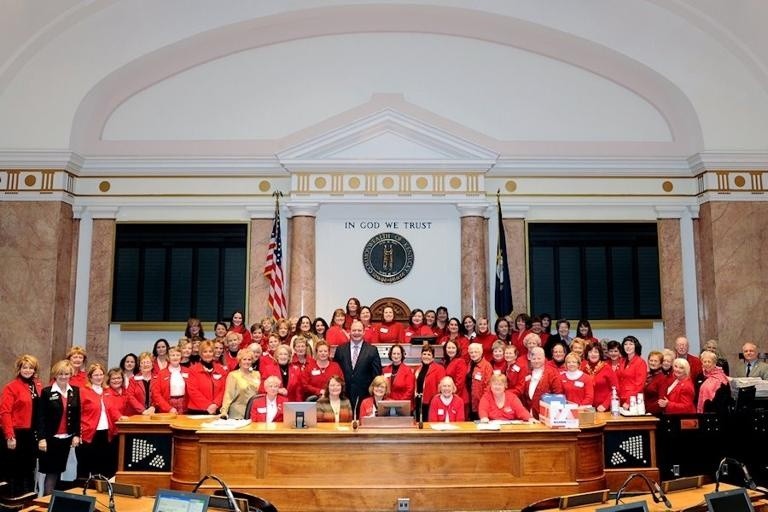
[532,481,767,511]
[20,484,241,512]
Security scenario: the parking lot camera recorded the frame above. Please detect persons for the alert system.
[2,347,134,501]
[120,297,622,426]
[608,318,768,482]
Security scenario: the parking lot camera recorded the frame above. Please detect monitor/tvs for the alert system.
[283,402,317,429]
[411,336,437,345]
[378,400,410,416]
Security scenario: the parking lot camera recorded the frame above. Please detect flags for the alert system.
[264,197,288,319]
[494,194,513,318]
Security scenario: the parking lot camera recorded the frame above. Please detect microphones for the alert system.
[107,484,115,508]
[419,395,423,428]
[224,485,242,512]
[741,464,757,491]
[352,396,360,432]
[652,480,673,508]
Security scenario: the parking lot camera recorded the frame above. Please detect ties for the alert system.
[746,361,752,378]
[352,345,358,370]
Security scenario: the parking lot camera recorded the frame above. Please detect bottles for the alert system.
[629,392,644,416]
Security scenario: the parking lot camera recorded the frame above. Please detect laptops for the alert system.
[704,487,754,512]
[47,490,96,512]
[152,489,210,512]
[596,500,648,512]
